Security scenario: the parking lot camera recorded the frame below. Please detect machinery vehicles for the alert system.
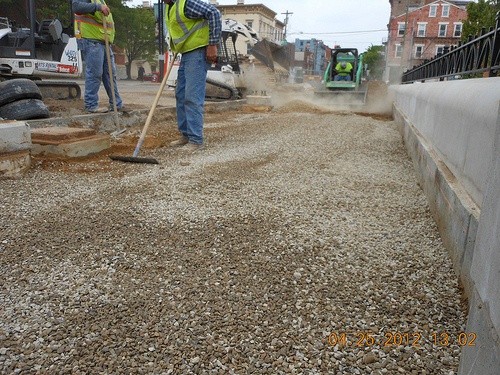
[164,16,292,102]
[0,0,83,101]
[315,48,368,112]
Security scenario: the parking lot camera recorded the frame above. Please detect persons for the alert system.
[162,0,222,152]
[137,64,146,82]
[333,58,354,83]
[72,0,132,113]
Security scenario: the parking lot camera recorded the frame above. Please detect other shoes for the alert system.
[182,142,202,151]
[92,106,108,113]
[170,135,189,146]
[118,105,133,112]
